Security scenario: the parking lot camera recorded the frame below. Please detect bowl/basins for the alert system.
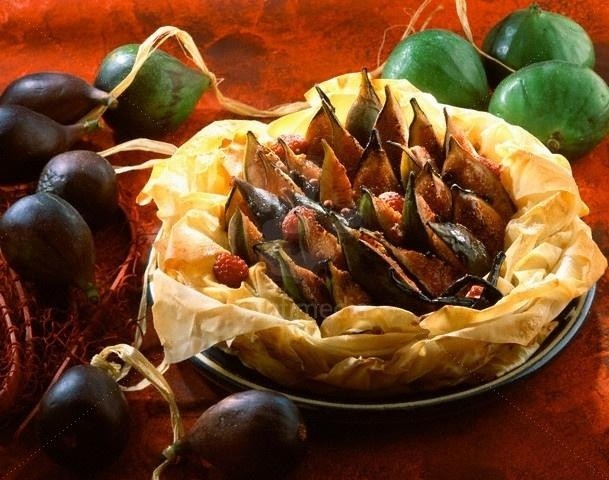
[139,221,597,417]
